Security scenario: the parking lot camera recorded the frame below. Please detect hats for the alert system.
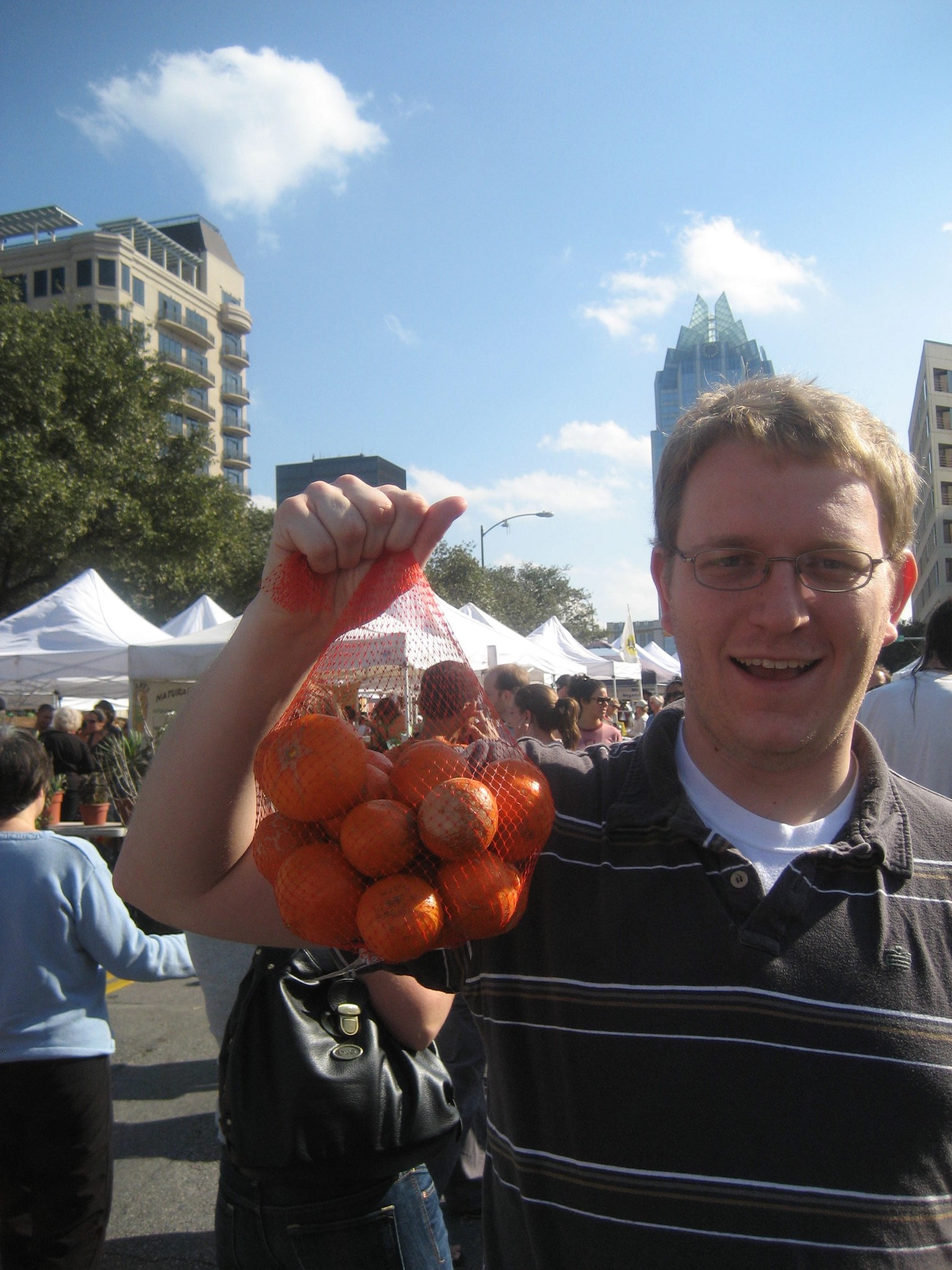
[636,700,648,707]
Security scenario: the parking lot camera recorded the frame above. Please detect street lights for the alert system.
[479,511,555,568]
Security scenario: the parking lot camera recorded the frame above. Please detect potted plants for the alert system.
[78,772,110,826]
[95,725,167,827]
[41,773,67,828]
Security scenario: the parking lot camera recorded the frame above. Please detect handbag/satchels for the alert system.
[218,946,461,1187]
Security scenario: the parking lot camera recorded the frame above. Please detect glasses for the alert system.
[85,719,101,725]
[660,535,891,590]
[556,686,564,693]
[665,691,685,701]
[586,695,611,704]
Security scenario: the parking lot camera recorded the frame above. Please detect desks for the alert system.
[44,821,128,859]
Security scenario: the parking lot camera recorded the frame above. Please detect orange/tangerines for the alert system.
[252,714,552,962]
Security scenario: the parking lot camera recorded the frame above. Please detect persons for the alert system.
[113,372,952,1270]
[858,597,952,798]
[0,660,686,823]
[213,944,489,1269]
[0,722,194,1270]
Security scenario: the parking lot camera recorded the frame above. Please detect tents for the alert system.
[1,566,681,732]
